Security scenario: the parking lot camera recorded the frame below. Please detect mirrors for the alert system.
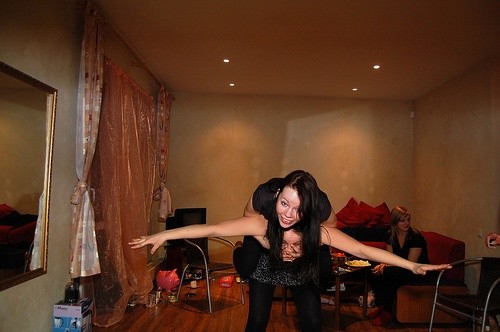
[0,62,56,291]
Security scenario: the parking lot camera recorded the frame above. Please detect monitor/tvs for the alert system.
[166,208,206,246]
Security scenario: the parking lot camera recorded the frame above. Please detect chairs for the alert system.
[428,255,500,332]
[172,208,245,313]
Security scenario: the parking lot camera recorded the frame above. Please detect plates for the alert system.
[345,261,371,267]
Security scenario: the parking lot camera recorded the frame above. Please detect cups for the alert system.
[64,282,78,303]
[191,281,196,287]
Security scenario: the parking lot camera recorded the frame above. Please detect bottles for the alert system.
[156,287,163,304]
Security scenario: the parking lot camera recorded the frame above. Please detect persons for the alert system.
[128,206,452,332]
[368,206,434,328]
[232,170,337,290]
[484,232,500,250]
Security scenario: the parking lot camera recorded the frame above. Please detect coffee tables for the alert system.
[282,264,370,332]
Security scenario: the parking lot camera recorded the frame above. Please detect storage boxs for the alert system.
[52,297,93,332]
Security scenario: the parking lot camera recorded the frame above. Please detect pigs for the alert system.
[156,268,180,293]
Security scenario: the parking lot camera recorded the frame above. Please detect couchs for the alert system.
[0,203,38,248]
[330,231,470,325]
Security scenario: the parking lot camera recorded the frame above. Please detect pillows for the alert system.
[357,201,384,230]
[373,201,392,231]
[336,197,371,230]
